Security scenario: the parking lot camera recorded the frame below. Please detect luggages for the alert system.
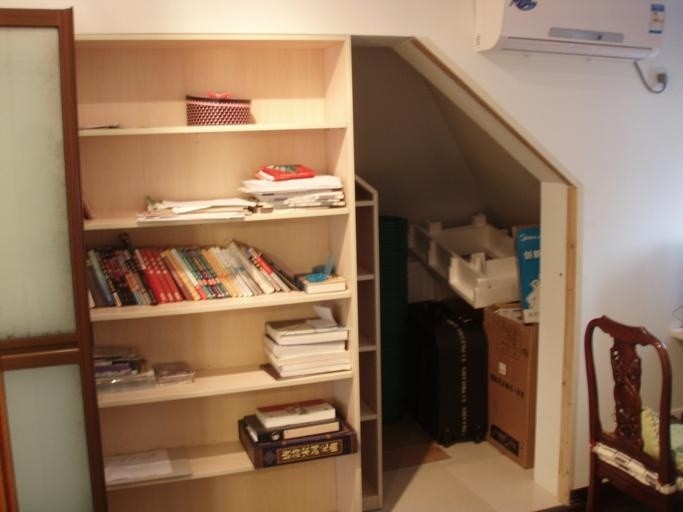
[402,300,492,446]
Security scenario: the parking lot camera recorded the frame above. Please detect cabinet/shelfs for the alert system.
[77,32,383,511]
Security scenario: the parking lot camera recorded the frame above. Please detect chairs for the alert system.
[582,314,683,512]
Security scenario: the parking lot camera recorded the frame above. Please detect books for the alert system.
[264,317,351,379]
[92,345,195,389]
[237,399,354,468]
[88,239,300,308]
[136,165,345,222]
[295,272,346,294]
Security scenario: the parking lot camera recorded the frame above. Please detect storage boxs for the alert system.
[484,300,539,471]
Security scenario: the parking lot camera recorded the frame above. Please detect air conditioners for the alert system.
[474,1,668,62]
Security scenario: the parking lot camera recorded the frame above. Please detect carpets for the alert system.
[381,433,452,469]
